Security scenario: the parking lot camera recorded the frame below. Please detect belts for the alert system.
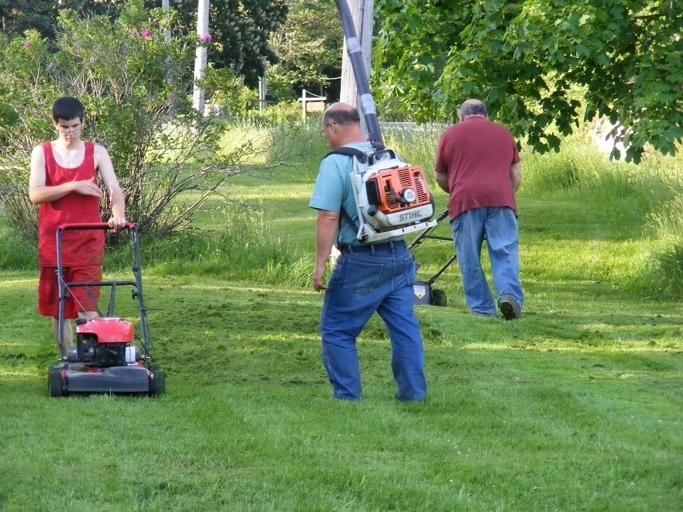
[336,237,407,255]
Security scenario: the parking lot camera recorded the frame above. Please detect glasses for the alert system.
[320,122,338,137]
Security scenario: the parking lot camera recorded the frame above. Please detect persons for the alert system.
[433,99,524,320]
[29,98,127,360]
[308,102,428,401]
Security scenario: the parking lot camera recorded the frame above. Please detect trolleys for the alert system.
[46,220,168,397]
[406,203,458,309]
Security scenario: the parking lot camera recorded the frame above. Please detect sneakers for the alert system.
[499,294,521,322]
